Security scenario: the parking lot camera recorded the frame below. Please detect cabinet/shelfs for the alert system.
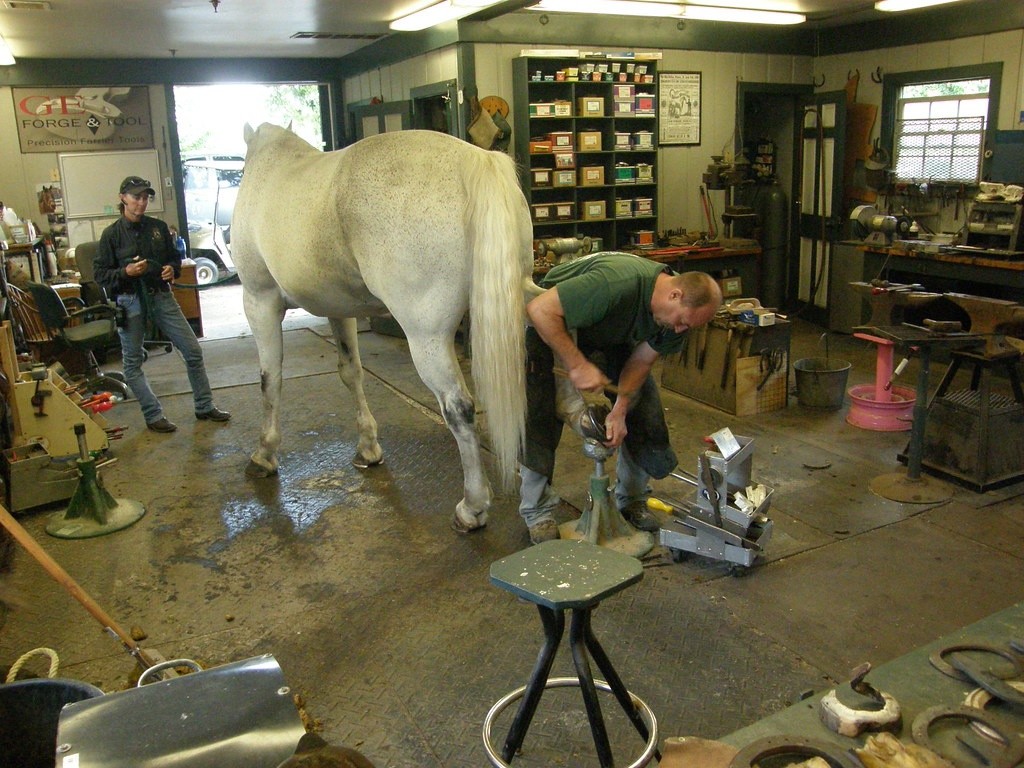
[510,54,662,254]
[173,257,204,337]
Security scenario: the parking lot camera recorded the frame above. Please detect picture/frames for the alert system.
[656,70,702,145]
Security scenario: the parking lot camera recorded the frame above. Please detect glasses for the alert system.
[122,179,151,191]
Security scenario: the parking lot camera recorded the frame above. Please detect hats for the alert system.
[120,176,155,196]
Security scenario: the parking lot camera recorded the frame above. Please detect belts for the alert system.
[118,287,166,295]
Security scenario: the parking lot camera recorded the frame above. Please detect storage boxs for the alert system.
[529,83,656,245]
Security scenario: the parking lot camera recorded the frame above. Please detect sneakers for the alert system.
[528,519,561,545]
[146,417,178,433]
[618,500,659,531]
[195,408,231,421]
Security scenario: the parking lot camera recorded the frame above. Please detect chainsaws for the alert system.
[850,205,910,246]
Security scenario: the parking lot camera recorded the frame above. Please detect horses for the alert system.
[228,117,532,534]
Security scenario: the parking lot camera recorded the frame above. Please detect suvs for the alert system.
[182,149,248,285]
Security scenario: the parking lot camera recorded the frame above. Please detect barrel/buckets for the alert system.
[793,333,851,411]
[0,677,105,768]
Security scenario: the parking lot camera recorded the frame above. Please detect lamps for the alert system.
[876,0,961,11]
[389,1,507,31]
[529,1,806,25]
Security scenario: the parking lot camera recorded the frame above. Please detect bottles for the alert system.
[176,236,186,259]
[910,221,918,240]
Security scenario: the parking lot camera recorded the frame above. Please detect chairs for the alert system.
[26,280,131,401]
[5,283,101,373]
[75,240,173,362]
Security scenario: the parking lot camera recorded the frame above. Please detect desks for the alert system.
[3,235,44,286]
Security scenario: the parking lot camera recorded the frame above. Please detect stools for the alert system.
[482,538,664,767]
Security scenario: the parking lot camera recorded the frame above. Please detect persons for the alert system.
[515,252,724,544]
[94,176,231,434]
[169,225,187,259]
